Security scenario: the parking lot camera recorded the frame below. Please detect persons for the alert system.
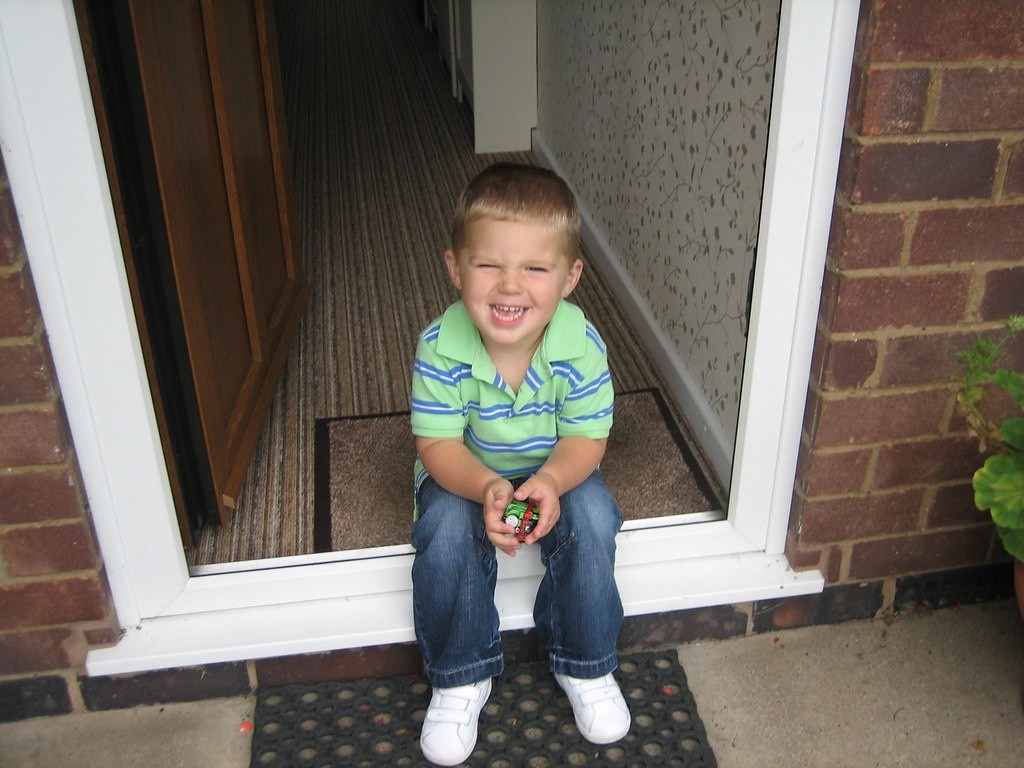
[407,160,632,766]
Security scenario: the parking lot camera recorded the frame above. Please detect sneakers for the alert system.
[419,676,492,767]
[553,671,632,745]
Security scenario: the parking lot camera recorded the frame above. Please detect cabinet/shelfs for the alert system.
[424,0,537,155]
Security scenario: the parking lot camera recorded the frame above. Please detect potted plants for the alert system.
[949,313,1024,614]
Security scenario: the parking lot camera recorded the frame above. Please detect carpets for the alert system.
[313,386,722,554]
[249,649,718,768]
